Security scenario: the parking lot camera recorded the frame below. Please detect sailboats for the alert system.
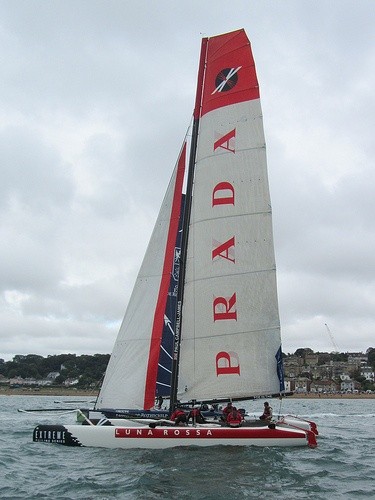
[31,28,319,448]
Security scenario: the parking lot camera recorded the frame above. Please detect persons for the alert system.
[187,408,206,425]
[259,402,273,421]
[170,409,188,425]
[223,403,237,415]
[226,407,242,427]
[155,396,163,410]
